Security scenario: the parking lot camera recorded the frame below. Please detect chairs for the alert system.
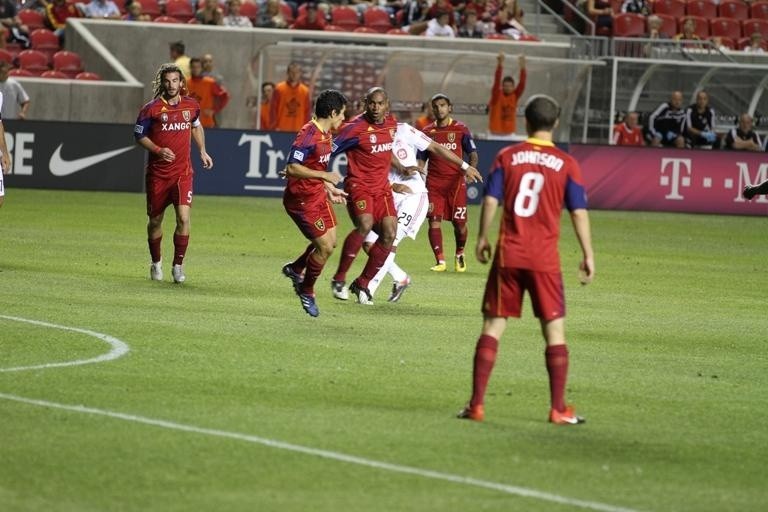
[0,1,767,93]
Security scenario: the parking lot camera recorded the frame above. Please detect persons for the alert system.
[133,61,213,284]
[742,177,768,200]
[458,93,598,427]
[279,87,484,303]
[277,89,350,318]
[1,63,30,198]
[165,40,313,132]
[484,50,527,136]
[611,91,761,153]
[1,0,543,55]
[569,1,765,61]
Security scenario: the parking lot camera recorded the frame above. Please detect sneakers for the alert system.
[549,407,585,424]
[171,264,185,284]
[295,281,319,316]
[334,275,410,304]
[283,262,305,290]
[431,262,448,271]
[454,255,466,273]
[150,256,164,281]
[456,402,485,419]
